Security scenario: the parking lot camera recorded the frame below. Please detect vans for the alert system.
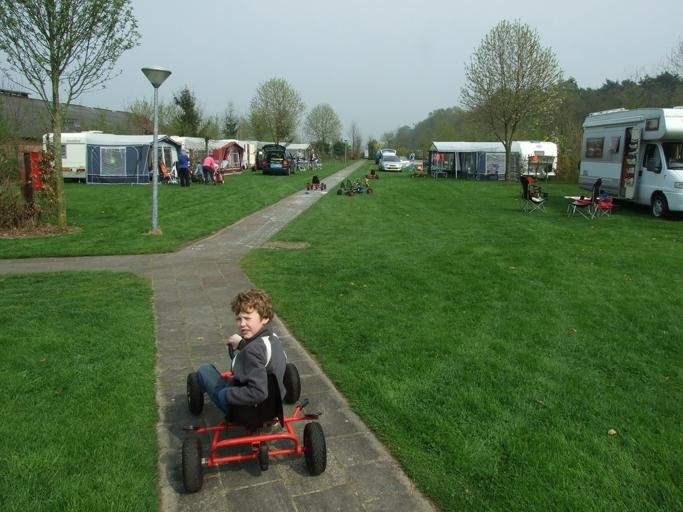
[579,110,683,218]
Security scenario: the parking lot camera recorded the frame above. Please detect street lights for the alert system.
[141,66,172,235]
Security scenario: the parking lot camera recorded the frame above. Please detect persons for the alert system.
[520,175,535,200]
[406,151,444,178]
[291,146,321,172]
[196,288,287,427]
[170,149,226,187]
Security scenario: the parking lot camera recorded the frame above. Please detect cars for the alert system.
[378,149,403,172]
[262,143,295,176]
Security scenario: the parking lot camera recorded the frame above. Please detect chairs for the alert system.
[520,175,614,220]
[160,161,178,184]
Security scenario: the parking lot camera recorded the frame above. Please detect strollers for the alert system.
[213,168,224,185]
[159,160,178,185]
